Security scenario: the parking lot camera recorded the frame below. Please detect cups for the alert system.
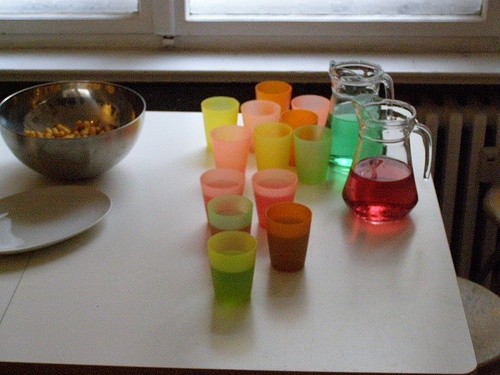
[207,195,253,237]
[265,200,312,273]
[255,80,291,111]
[211,128,250,170]
[291,95,330,127]
[293,126,332,185]
[202,97,239,150]
[241,100,281,152]
[252,122,293,170]
[282,110,318,167]
[206,230,255,310]
[252,168,298,229]
[200,169,246,216]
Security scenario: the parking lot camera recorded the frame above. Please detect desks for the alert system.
[0,109,477,375]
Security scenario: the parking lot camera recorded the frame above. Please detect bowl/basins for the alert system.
[0,81,146,183]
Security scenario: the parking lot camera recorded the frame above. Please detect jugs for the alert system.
[342,98,434,225]
[329,60,395,174]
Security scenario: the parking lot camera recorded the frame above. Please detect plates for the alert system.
[0,185,113,253]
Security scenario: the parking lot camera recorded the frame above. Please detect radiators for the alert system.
[415,104,500,279]
